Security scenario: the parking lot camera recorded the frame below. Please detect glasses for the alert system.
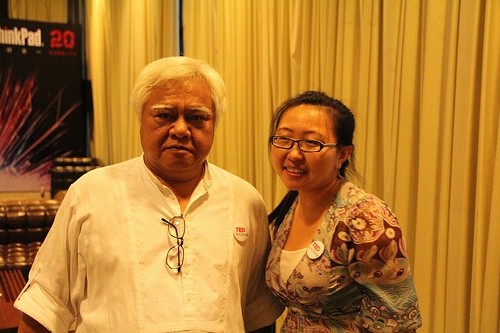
[162,214,186,273]
[270,136,338,152]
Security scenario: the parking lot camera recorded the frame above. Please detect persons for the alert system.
[265,90,423,333]
[13,56,286,333]
